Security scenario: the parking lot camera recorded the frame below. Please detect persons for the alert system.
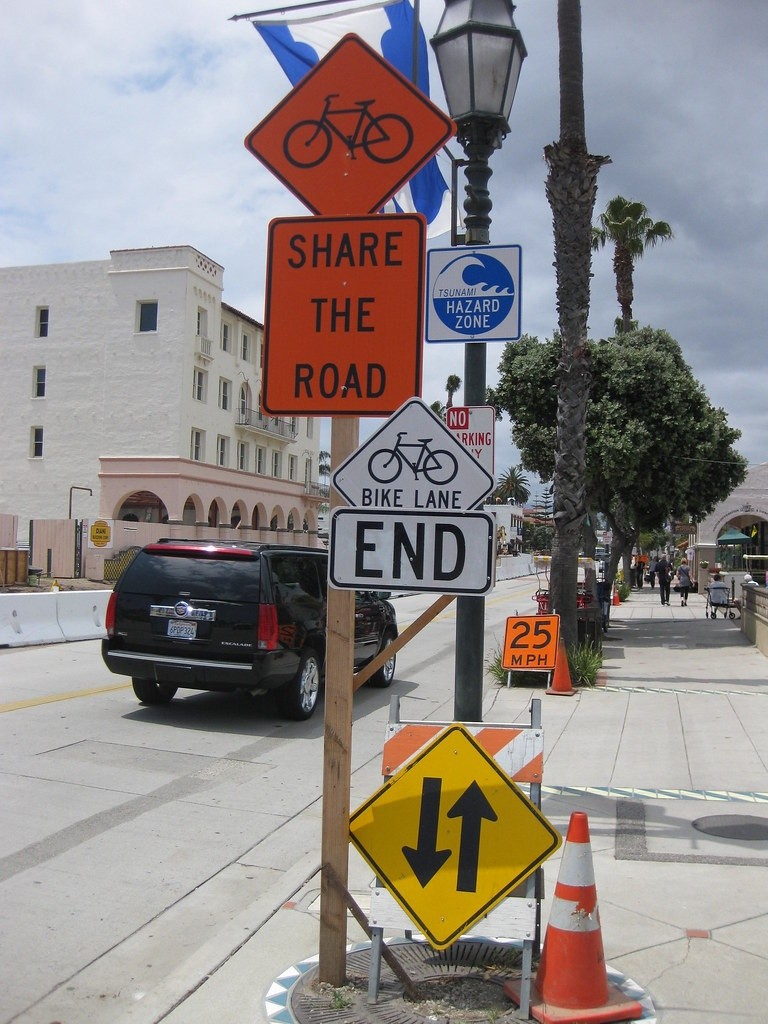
[648,557,658,590]
[634,555,645,590]
[709,574,729,603]
[655,554,674,606]
[676,557,691,606]
[733,573,759,620]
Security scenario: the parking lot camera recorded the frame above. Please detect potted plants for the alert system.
[617,582,632,602]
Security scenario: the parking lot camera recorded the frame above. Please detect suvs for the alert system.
[98,534,399,720]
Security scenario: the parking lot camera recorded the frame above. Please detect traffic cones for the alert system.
[613,589,622,606]
[499,809,644,1024]
[543,637,580,697]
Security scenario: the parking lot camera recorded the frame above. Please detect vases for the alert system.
[701,564,708,569]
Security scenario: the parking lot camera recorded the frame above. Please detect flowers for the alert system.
[699,560,710,565]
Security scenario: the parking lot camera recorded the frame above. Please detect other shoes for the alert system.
[667,601,670,606]
[662,602,664,605]
[685,602,687,606]
[681,600,684,606]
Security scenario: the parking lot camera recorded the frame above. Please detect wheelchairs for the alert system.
[703,586,736,620]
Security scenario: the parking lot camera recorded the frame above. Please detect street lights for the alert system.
[431,0,536,722]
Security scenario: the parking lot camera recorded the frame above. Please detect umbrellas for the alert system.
[717,528,751,555]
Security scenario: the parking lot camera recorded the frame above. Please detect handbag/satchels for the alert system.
[665,565,674,582]
[689,578,694,585]
